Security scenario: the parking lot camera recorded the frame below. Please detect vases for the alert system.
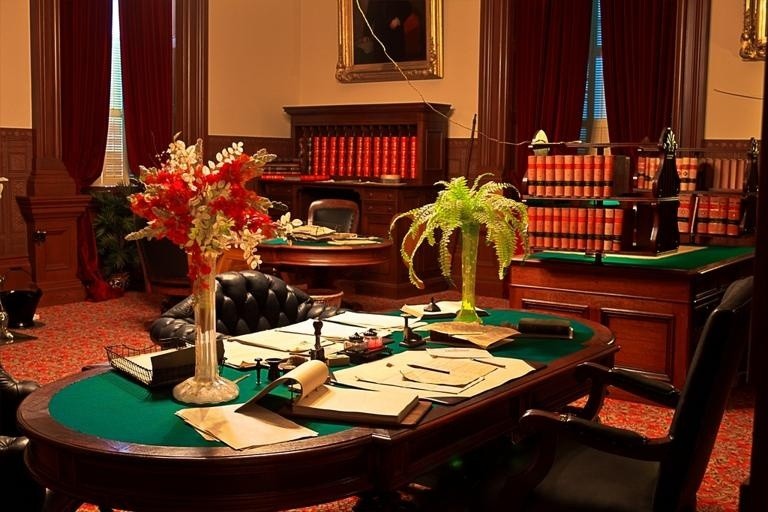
[173,252,240,405]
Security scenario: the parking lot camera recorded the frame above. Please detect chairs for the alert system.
[133,215,190,302]
[307,199,360,234]
[487,276,753,512]
[151,270,353,350]
[0,365,45,512]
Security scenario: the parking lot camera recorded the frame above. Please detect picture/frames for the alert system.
[740,0,768,62]
[336,0,444,84]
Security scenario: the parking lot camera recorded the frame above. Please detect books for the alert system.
[300,126,419,182]
[516,316,572,337]
[399,299,489,320]
[523,149,753,257]
[234,360,423,426]
[429,327,472,343]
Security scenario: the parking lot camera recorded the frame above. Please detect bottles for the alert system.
[345,330,383,353]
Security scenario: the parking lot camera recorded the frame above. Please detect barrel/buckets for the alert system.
[0,266,42,328]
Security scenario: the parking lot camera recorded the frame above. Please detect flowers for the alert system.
[127,130,303,310]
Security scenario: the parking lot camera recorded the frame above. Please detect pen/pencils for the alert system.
[232,374,251,384]
[473,359,505,367]
[408,364,450,374]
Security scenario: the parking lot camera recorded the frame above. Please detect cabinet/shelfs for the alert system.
[508,127,757,408]
[254,104,451,299]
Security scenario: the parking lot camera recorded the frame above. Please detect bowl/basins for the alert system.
[300,288,344,319]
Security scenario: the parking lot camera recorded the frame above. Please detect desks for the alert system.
[17,307,620,512]
[257,237,394,310]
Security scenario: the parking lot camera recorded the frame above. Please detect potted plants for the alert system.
[389,172,530,323]
[92,180,145,292]
